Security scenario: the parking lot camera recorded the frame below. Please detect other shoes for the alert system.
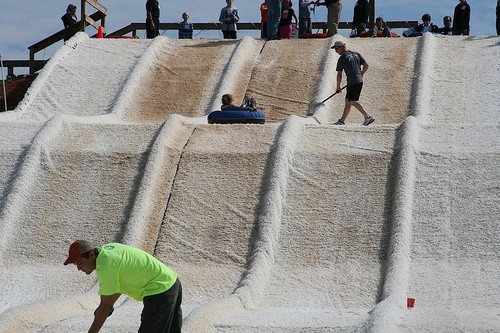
[331,119,345,125]
[361,116,375,126]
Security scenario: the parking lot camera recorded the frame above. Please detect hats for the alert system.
[442,16,452,21]
[63,239,96,266]
[330,40,346,49]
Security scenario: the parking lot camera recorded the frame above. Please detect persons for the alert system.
[495,0,500,36]
[350,0,370,36]
[452,0,471,36]
[219,0,239,39]
[437,16,453,35]
[316,0,343,37]
[178,12,193,39]
[64,240,183,333]
[145,0,160,39]
[330,41,375,126]
[60,4,78,29]
[298,0,316,36]
[260,0,299,40]
[373,16,385,36]
[220,93,257,112]
[415,13,439,33]
